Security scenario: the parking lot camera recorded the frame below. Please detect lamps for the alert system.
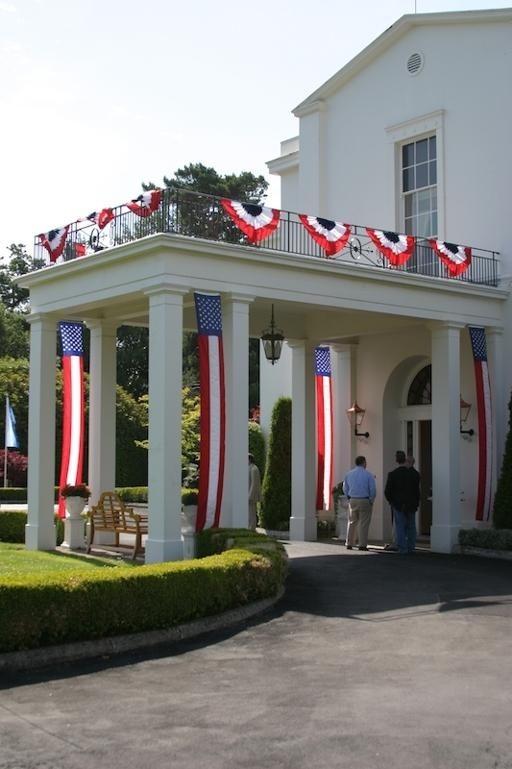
[258,299,286,365]
[345,398,369,439]
[456,393,474,437]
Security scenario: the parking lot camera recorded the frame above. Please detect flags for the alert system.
[6,398,21,449]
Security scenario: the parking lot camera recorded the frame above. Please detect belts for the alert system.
[350,497,368,499]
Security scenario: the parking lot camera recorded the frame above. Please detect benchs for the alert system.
[85,491,148,562]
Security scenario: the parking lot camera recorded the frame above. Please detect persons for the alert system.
[343,455,376,550]
[249,453,262,532]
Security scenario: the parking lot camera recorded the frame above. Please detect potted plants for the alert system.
[60,483,90,519]
[179,492,197,533]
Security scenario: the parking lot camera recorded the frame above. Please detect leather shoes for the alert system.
[346,546,352,550]
[386,545,399,551]
[359,546,368,551]
